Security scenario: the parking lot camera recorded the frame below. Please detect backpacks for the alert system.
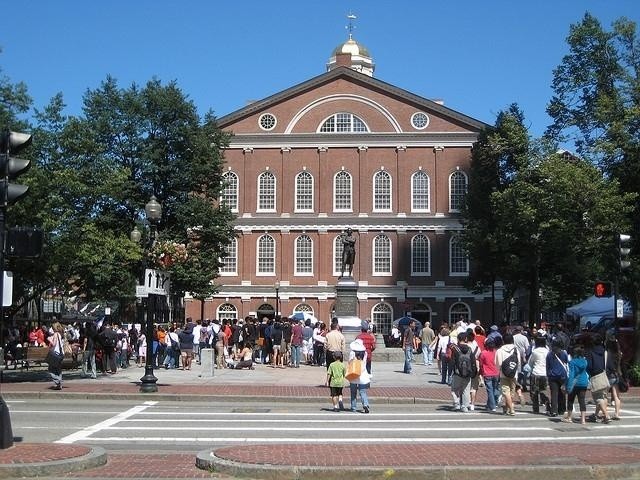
[454,345,472,377]
[212,327,220,349]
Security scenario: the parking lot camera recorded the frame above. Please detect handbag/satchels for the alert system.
[239,334,243,341]
[618,376,628,392]
[560,377,577,394]
[280,341,287,354]
[344,360,362,381]
[46,351,62,367]
[590,371,610,393]
[172,341,177,350]
[502,354,519,378]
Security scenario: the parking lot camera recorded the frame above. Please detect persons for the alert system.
[355,318,376,374]
[79,323,99,379]
[46,322,67,389]
[418,322,625,427]
[98,324,115,374]
[391,324,400,346]
[345,338,372,413]
[153,316,328,370]
[28,319,146,368]
[325,318,345,372]
[339,227,356,280]
[326,351,346,411]
[402,321,416,374]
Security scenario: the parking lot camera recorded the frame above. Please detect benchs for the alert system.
[20,346,79,371]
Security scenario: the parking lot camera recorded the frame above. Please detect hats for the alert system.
[350,338,366,352]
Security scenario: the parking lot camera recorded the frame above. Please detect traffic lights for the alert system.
[618,233,630,275]
[10,130,33,208]
[595,282,612,298]
[0,131,7,210]
[7,229,43,259]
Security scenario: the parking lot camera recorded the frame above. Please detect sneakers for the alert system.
[602,416,612,423]
[589,414,599,422]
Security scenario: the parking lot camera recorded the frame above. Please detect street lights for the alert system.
[130,224,160,253]
[508,297,515,324]
[403,283,409,316]
[274,281,281,317]
[140,194,162,392]
